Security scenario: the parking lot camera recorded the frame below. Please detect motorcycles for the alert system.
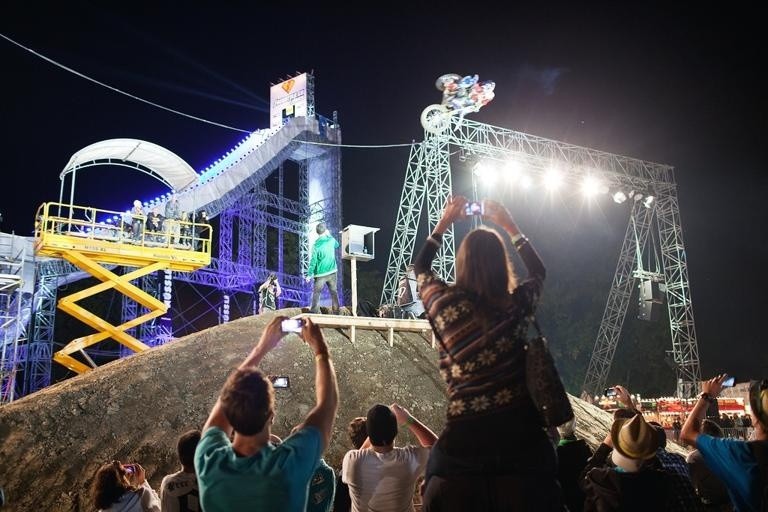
[419,71,480,136]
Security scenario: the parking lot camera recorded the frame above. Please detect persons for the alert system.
[555,373,768,512]
[130,199,145,240]
[269,401,442,512]
[450,80,497,133]
[411,192,569,512]
[195,209,211,248]
[305,223,341,314]
[92,459,162,512]
[146,207,166,235]
[190,313,342,512]
[161,429,204,512]
[164,194,183,244]
[257,272,282,314]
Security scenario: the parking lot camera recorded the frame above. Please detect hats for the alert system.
[366,404,397,438]
[611,412,659,460]
[749,380,768,426]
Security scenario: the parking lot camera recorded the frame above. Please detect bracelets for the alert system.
[315,353,329,361]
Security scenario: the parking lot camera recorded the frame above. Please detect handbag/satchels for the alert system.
[523,334,574,429]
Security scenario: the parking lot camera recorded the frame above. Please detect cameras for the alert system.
[721,376,736,388]
[270,375,290,391]
[280,320,305,335]
[603,388,617,398]
[120,464,134,475]
[457,200,487,218]
